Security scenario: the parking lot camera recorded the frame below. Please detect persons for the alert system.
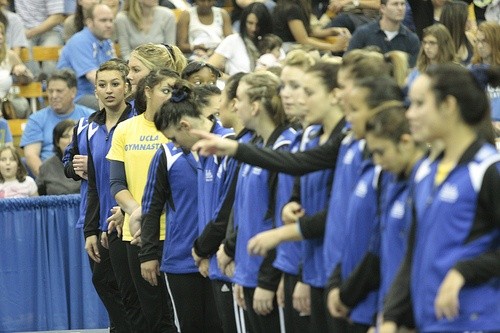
[0,0,500,333]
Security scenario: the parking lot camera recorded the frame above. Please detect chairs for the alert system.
[6,8,186,158]
